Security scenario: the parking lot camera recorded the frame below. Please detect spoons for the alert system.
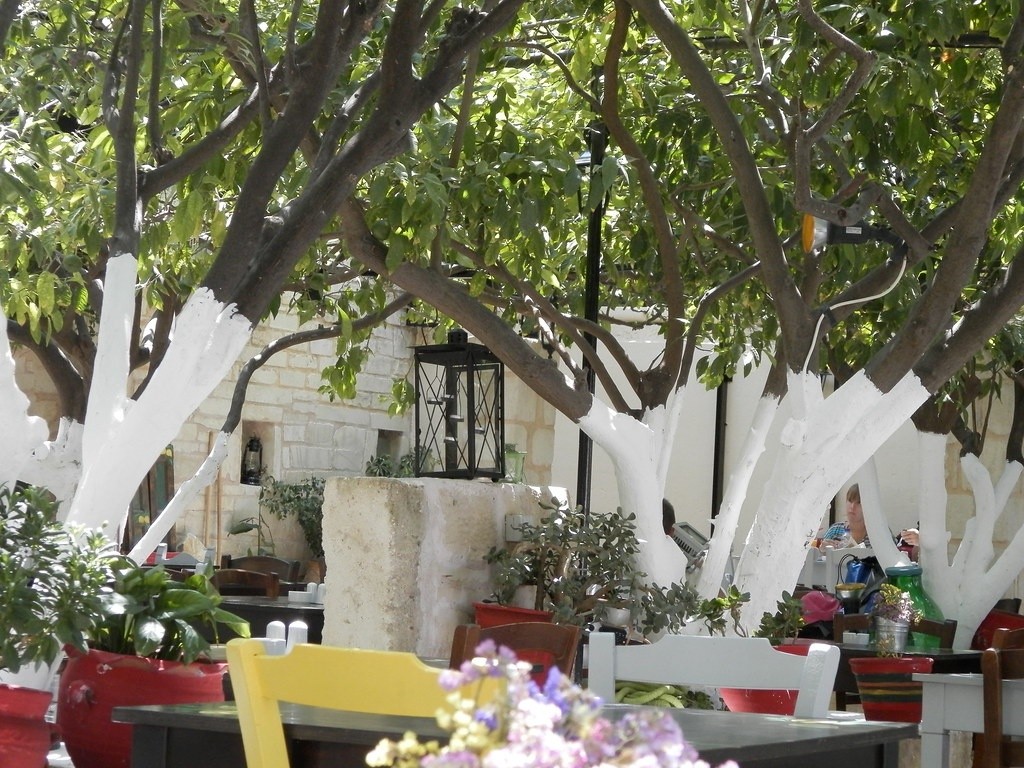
[897,537,903,547]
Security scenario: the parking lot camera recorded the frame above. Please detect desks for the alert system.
[802,641,983,690]
[113,696,918,767]
[183,580,324,645]
[913,671,1024,767]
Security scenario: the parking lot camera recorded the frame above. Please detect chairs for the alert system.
[227,636,508,768]
[588,630,840,718]
[967,593,1024,767]
[451,621,582,691]
[136,552,327,603]
[781,546,960,710]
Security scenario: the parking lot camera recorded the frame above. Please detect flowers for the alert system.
[363,637,740,768]
[868,581,923,625]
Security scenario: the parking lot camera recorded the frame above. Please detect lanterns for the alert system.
[496,440,528,483]
[241,434,263,486]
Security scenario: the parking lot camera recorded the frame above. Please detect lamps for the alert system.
[799,213,911,259]
[240,431,263,485]
[571,120,616,220]
[407,329,507,482]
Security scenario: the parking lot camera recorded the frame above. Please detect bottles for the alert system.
[812,584,827,592]
[816,527,825,547]
[844,528,851,539]
[885,566,944,647]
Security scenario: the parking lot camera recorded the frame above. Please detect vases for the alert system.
[877,619,909,645]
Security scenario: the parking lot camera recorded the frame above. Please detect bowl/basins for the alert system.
[288,591,312,603]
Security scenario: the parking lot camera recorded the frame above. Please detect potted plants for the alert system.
[57,554,250,768]
[849,635,934,721]
[472,498,703,693]
[701,586,830,714]
[0,482,116,768]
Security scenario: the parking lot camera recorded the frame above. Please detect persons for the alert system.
[900,528,922,547]
[818,481,872,556]
[661,497,687,596]
